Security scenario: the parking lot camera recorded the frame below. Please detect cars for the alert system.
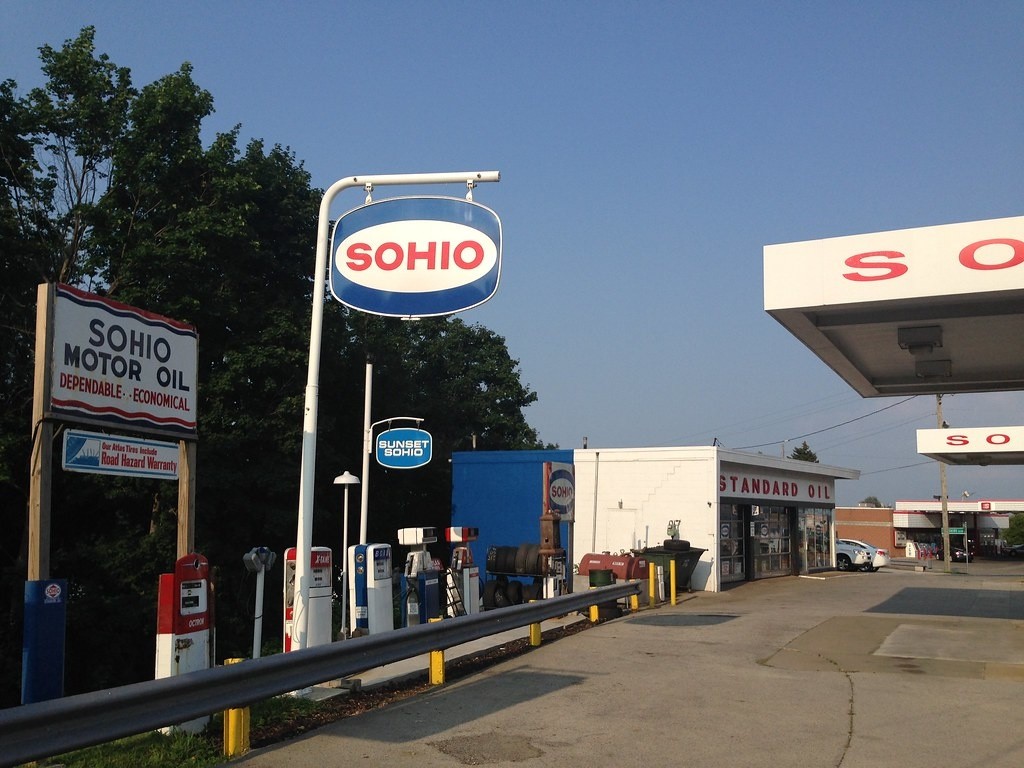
[1002,544,1024,558]
[839,538,890,572]
[836,538,868,571]
[935,547,974,563]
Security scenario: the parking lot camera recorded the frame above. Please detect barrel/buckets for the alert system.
[589,569,617,608]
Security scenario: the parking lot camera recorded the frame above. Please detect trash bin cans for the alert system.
[631,545,709,593]
